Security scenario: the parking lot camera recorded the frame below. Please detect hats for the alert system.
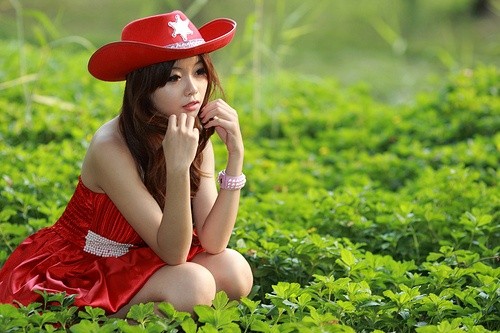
[87,10,238,82]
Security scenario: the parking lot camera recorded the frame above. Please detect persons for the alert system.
[0,10,254,331]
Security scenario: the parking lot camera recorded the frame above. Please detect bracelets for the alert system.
[218,169,247,190]
